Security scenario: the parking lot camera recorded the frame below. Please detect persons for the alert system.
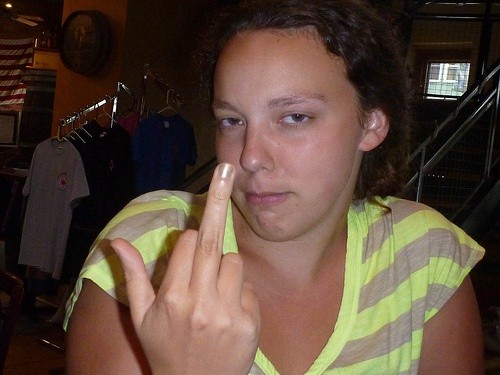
[65,0,489,375]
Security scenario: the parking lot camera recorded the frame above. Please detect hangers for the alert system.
[65,115,74,141]
[53,117,67,145]
[158,88,179,118]
[75,112,92,138]
[97,95,117,122]
[68,112,86,144]
